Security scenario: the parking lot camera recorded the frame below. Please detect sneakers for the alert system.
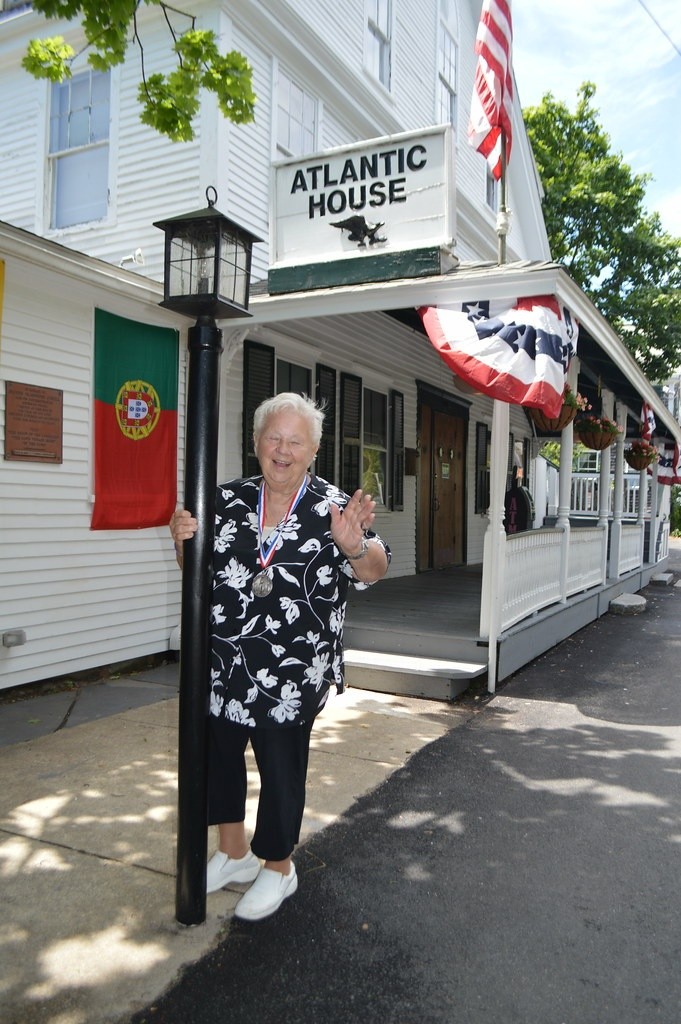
[207,850,260,894]
[235,860,298,920]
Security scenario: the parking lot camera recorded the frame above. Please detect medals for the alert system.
[248,574,275,599]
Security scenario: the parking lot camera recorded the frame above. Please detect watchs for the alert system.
[339,537,369,562]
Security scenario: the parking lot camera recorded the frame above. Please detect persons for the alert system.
[169,391,393,923]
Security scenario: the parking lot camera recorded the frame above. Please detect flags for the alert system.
[466,0,514,181]
[90,305,181,531]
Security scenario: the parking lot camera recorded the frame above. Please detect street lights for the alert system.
[150,181,266,935]
